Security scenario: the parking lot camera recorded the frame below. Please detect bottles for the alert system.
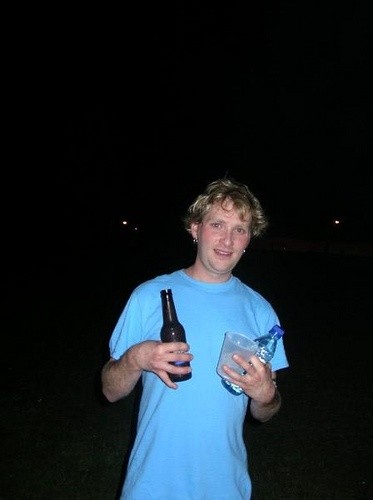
[160,289,192,383]
[221,325,285,396]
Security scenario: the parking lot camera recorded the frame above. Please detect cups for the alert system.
[216,332,258,379]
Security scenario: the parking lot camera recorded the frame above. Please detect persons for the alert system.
[100,180,291,500]
